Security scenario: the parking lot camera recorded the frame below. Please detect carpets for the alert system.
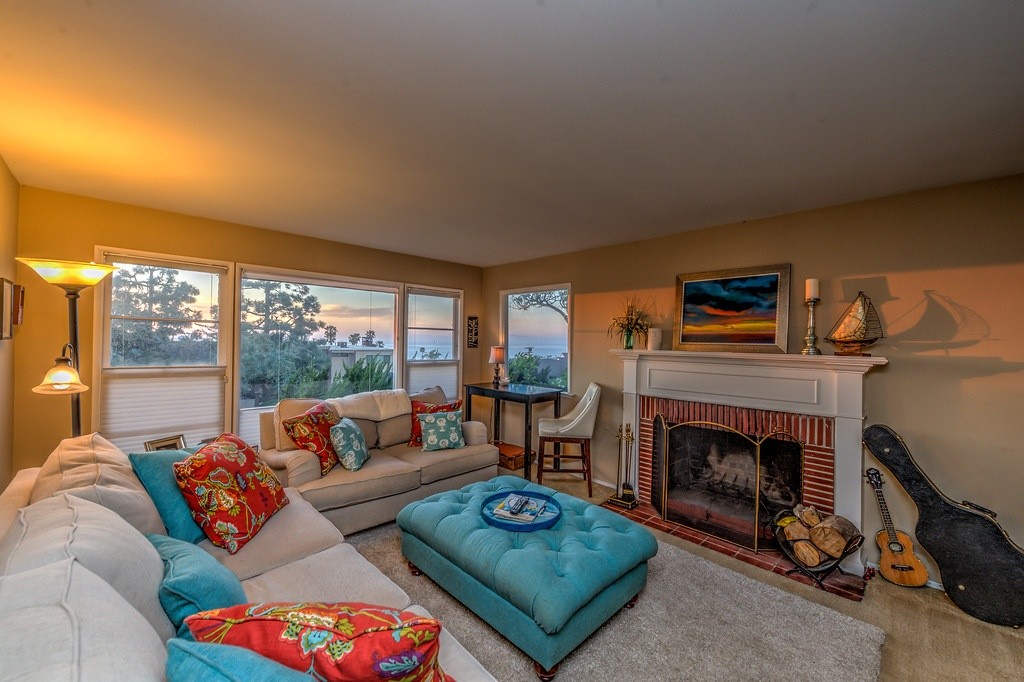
[343,520,886,682]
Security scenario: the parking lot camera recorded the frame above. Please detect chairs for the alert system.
[535,382,601,499]
[487,344,508,390]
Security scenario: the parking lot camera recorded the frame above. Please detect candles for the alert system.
[805,278,820,299]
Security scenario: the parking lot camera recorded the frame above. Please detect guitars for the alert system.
[862,467,929,587]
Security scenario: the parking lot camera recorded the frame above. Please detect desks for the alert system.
[462,381,562,482]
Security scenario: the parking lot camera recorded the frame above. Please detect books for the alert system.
[493,493,546,523]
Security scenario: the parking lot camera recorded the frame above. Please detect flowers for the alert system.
[606,295,657,349]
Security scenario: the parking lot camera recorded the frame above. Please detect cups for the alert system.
[648,328,662,350]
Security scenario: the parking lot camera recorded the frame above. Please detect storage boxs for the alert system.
[494,443,537,471]
[498,379,510,386]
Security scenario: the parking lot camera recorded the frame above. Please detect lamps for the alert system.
[14,255,122,441]
[31,343,90,396]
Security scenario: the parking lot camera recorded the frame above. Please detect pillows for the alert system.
[144,532,250,643]
[183,598,459,682]
[417,410,468,452]
[407,400,463,449]
[172,432,290,555]
[278,401,341,476]
[329,416,371,472]
[166,636,321,682]
[128,446,209,544]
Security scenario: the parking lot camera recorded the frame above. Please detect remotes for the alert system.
[510,497,529,515]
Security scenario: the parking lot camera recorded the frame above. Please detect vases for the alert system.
[623,331,633,349]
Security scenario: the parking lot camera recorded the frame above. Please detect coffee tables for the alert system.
[393,471,659,682]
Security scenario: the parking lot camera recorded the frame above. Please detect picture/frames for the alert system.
[671,262,792,355]
[144,435,187,452]
[0,277,15,341]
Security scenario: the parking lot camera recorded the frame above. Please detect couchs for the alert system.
[0,431,500,682]
[256,385,502,537]
